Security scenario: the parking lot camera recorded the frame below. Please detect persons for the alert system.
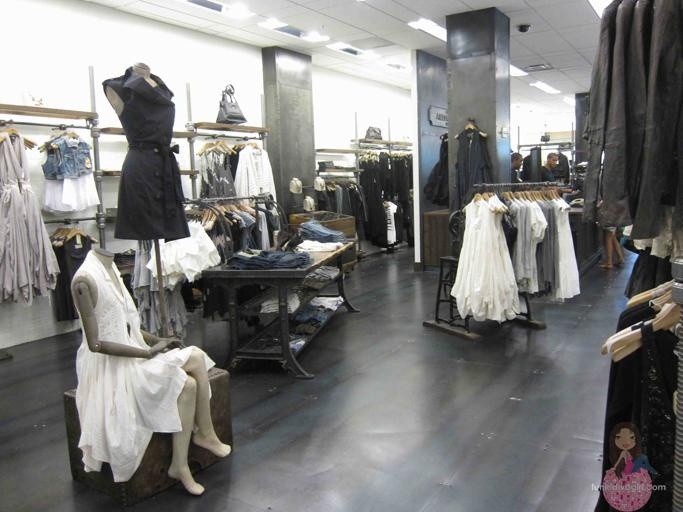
[597,225,626,269]
[71,248,232,496]
[102,63,191,243]
[540,152,559,182]
[511,152,523,183]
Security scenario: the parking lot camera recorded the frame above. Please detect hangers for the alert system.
[321,174,358,193]
[600,278,683,365]
[46,219,100,250]
[0,120,93,155]
[462,180,571,222]
[196,139,263,159]
[359,149,411,167]
[454,118,487,140]
[184,196,260,232]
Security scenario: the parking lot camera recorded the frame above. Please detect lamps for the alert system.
[289,174,329,224]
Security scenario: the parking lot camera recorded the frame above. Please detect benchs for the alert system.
[64,366,230,506]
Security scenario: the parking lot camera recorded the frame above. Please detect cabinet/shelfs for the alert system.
[316,146,365,177]
[97,123,199,179]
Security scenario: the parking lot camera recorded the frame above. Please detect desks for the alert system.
[199,241,356,380]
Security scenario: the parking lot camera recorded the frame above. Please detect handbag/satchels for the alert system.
[216,84,247,124]
[365,126,382,140]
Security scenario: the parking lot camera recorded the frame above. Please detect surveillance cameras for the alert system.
[518,24,531,32]
